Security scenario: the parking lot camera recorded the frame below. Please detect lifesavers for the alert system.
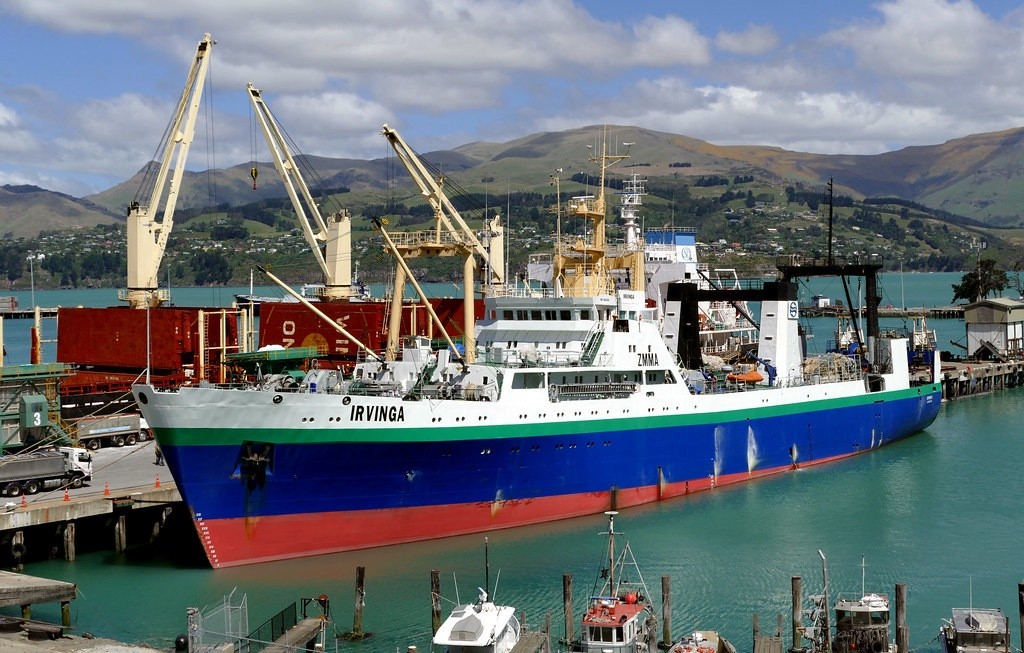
[698,645,715,653]
[675,645,694,653]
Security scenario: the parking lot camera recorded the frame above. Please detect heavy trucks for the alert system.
[0,447,94,499]
[75,411,150,450]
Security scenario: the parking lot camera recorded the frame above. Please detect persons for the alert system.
[155,443,165,467]
[542,281,548,297]
[519,273,525,283]
[704,370,745,393]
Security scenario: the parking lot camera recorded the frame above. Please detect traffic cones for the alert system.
[102,481,112,495]
[154,473,161,488]
[21,493,28,508]
[63,488,70,501]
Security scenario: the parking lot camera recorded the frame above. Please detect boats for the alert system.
[428,535,521,653]
[566,510,656,653]
[938,575,1012,653]
[791,548,891,653]
[667,630,737,653]
[129,123,944,571]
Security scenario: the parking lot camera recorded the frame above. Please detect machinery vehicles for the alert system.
[54,34,504,411]
